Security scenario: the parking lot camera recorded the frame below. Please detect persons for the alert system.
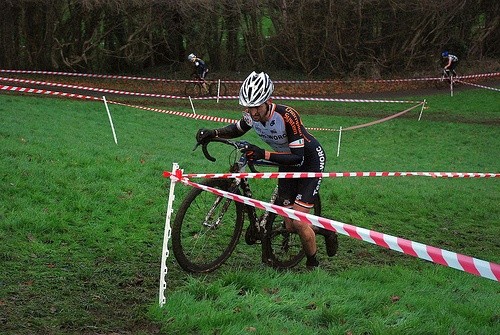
[188,54,209,94]
[441,51,458,76]
[197,73,326,271]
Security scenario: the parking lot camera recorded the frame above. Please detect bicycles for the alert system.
[435,66,460,89]
[182,72,227,98]
[173,136,318,273]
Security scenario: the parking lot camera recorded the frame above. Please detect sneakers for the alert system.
[306,259,319,272]
[324,232,339,257]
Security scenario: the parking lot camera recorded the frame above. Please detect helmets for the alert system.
[442,51,448,57]
[188,54,195,60]
[238,71,275,107]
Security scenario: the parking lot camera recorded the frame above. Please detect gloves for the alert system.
[196,128,217,144]
[240,144,265,160]
